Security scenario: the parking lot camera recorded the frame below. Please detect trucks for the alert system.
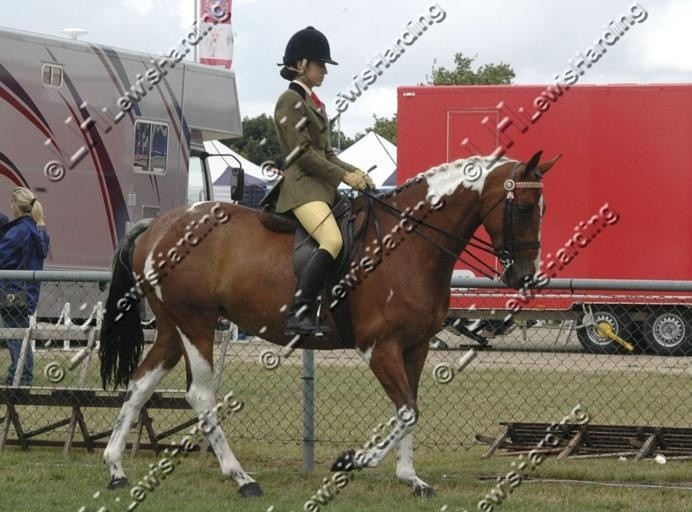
[1,23,245,331]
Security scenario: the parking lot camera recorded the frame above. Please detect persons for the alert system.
[0,186,49,386]
[274,26,376,335]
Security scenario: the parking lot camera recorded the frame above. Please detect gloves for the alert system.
[341,168,376,192]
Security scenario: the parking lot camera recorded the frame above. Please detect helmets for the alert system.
[276,26,339,66]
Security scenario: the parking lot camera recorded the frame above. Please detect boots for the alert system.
[284,249,337,337]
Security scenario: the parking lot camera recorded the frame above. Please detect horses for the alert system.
[97,147,565,501]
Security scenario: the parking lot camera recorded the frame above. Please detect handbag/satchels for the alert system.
[0,283,27,315]
